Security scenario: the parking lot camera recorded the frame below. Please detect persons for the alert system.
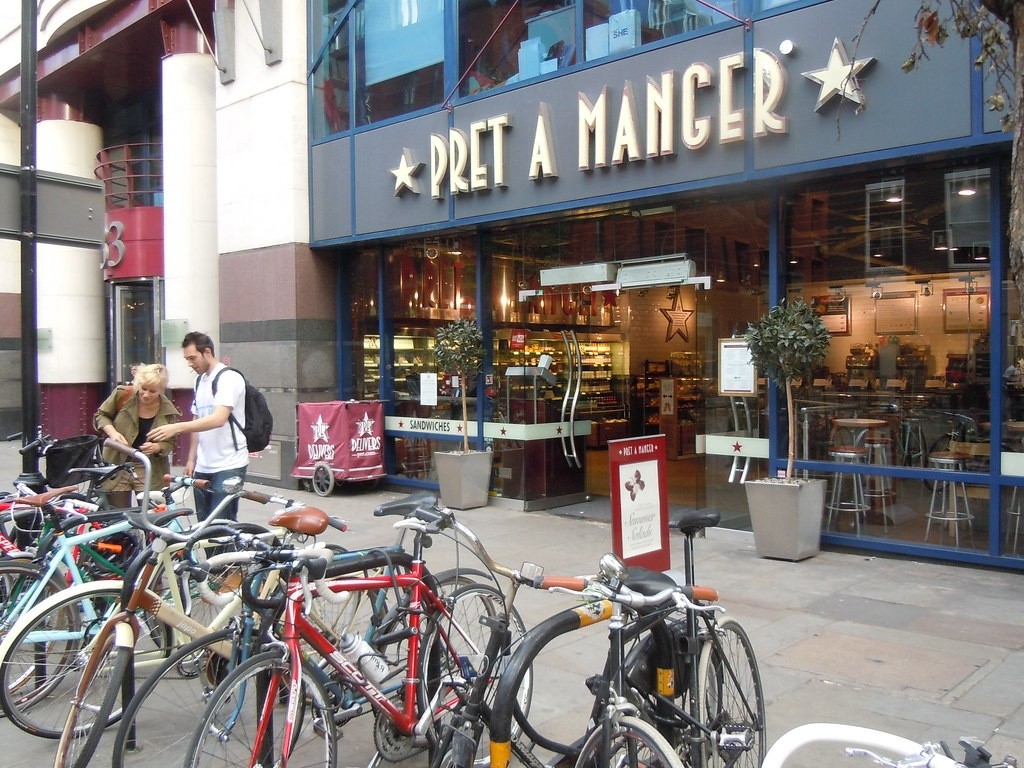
[93,364,181,546]
[146,331,250,579]
[128,361,139,385]
[1003,358,1024,420]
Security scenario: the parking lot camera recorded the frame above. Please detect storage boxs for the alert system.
[587,419,628,448]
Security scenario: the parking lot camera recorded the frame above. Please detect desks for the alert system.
[832,418,887,523]
[981,421,1024,432]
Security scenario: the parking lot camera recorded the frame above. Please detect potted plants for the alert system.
[434,319,494,510]
[743,296,830,560]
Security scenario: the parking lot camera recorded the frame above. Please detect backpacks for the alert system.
[194,368,273,451]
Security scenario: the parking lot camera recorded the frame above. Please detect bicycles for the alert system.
[0,429,767,767]
[732,384,1014,494]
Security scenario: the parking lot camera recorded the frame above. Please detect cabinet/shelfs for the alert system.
[630,360,718,437]
[360,331,626,417]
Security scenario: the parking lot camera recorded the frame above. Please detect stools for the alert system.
[826,414,1024,555]
